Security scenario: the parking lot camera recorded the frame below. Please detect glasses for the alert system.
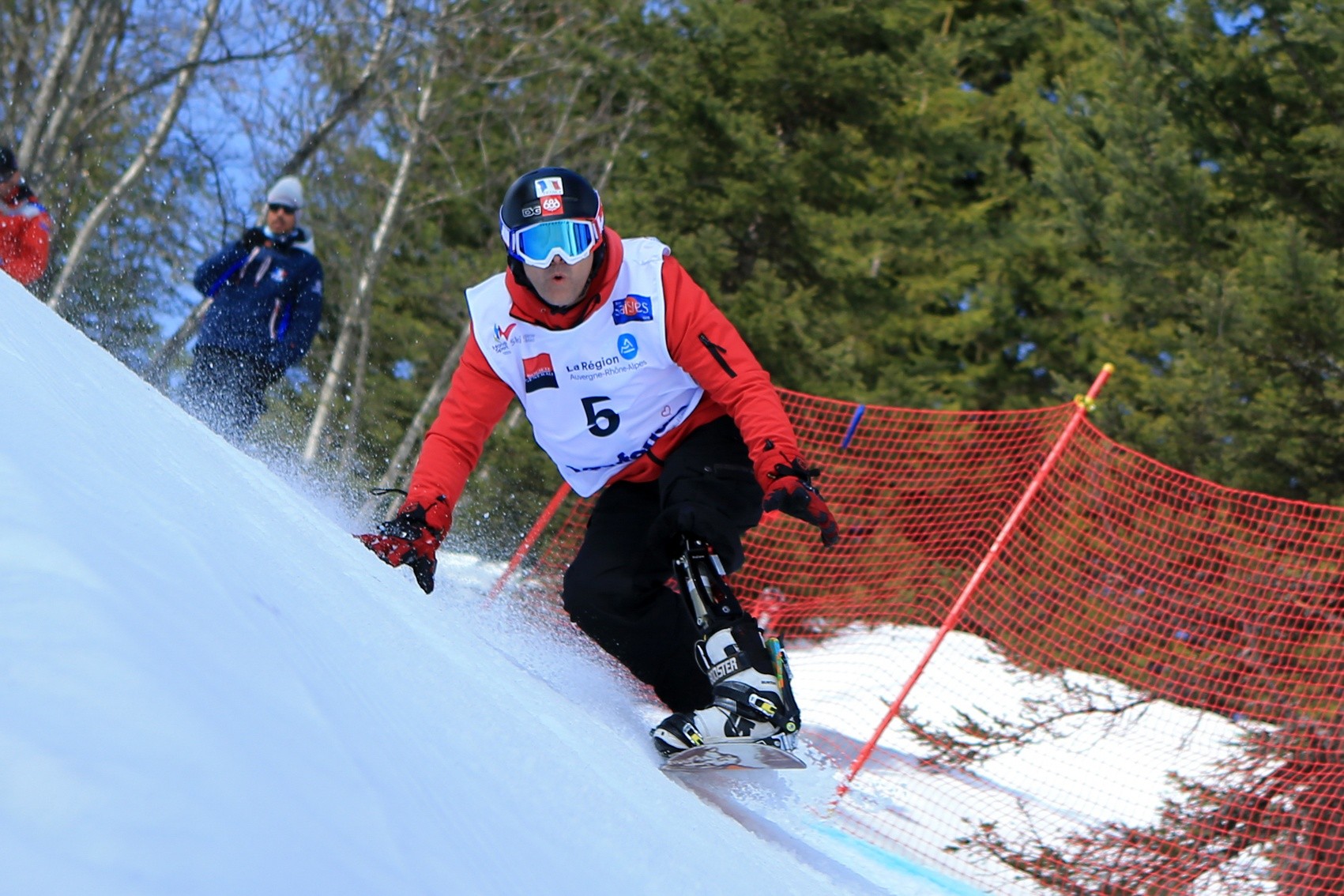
[266,203,297,213]
[497,208,606,269]
[0,171,14,184]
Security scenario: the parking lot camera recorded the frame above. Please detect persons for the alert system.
[0,143,53,284]
[177,176,323,454]
[350,167,838,757]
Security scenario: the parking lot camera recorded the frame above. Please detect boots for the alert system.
[643,624,802,756]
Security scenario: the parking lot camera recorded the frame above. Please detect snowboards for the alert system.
[659,742,807,773]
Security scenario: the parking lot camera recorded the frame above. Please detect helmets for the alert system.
[497,165,613,283]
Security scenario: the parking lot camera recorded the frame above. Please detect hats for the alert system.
[267,175,307,211]
[0,146,18,171]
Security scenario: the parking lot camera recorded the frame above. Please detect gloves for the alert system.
[762,470,850,549]
[258,357,290,384]
[242,225,272,253]
[356,506,442,595]
